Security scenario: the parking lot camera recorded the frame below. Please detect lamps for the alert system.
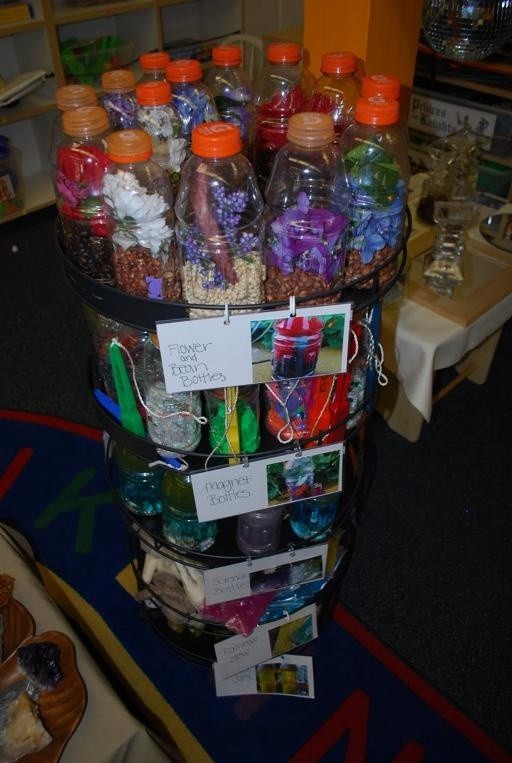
[421,0,512,61]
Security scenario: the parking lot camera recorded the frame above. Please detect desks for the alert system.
[374,172,512,443]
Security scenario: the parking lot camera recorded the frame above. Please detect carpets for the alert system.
[1,411,511,759]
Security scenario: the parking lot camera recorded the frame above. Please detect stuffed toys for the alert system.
[140,528,205,609]
[132,570,208,640]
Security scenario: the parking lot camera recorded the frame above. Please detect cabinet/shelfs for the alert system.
[53,208,414,656]
[0,1,245,225]
[408,45,512,207]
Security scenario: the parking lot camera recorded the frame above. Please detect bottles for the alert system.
[52,38,406,316]
[94,320,264,453]
[116,443,165,520]
[162,464,216,552]
[290,490,340,538]
[416,134,481,225]
[233,506,283,558]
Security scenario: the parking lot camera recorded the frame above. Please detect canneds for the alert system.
[271,318,325,381]
